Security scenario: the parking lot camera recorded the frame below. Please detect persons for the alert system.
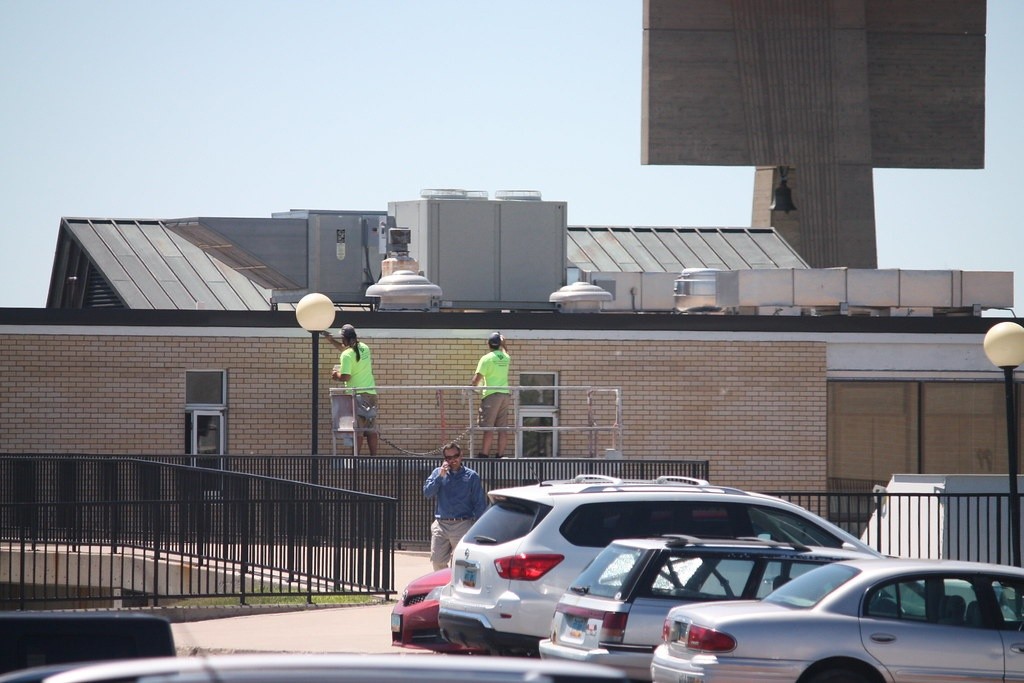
[423,442,487,572]
[319,323,378,456]
[469,331,510,459]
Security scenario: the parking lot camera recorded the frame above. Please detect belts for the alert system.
[441,517,468,520]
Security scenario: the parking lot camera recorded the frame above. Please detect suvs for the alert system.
[0,654,641,683]
[438,475,983,683]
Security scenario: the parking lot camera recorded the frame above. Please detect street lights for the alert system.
[296,292,336,546]
[983,321,1024,564]
[534,374,553,457]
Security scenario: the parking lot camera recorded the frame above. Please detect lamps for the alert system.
[839,302,849,315]
[873,484,885,504]
[971,304,981,316]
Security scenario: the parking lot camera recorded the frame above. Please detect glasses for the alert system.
[446,452,460,460]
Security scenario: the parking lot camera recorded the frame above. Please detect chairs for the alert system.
[939,595,966,621]
[772,576,792,589]
[966,600,984,623]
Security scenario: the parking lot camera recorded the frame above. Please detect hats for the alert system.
[488,332,500,346]
[340,323,355,337]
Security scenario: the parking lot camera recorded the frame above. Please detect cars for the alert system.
[649,556,1024,683]
[388,563,484,653]
